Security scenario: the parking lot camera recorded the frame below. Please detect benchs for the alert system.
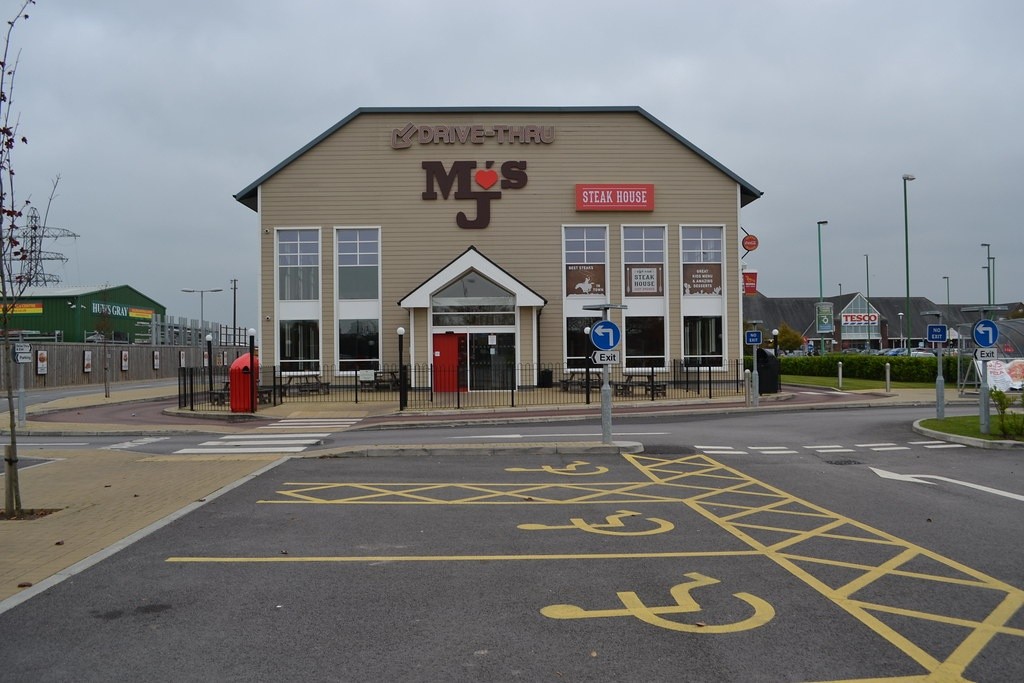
[559,379,605,387]
[361,378,410,390]
[644,384,666,397]
[613,383,638,397]
[283,382,330,397]
[207,390,230,405]
[258,388,272,405]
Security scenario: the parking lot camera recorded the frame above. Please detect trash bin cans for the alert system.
[756,348,778,395]
[541,368,553,387]
[229,353,259,413]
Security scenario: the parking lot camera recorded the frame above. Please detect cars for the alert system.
[843,347,977,357]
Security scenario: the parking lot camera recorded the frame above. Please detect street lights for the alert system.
[902,174,915,357]
[980,243,995,320]
[942,276,949,355]
[817,220,828,355]
[864,254,870,356]
[181,289,223,385]
[838,283,842,349]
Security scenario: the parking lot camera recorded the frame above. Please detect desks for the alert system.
[286,374,321,396]
[222,381,267,405]
[619,373,659,398]
[562,372,602,392]
[370,371,399,392]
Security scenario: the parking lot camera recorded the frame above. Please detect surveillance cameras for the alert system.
[266,316,270,320]
[265,229,269,233]
